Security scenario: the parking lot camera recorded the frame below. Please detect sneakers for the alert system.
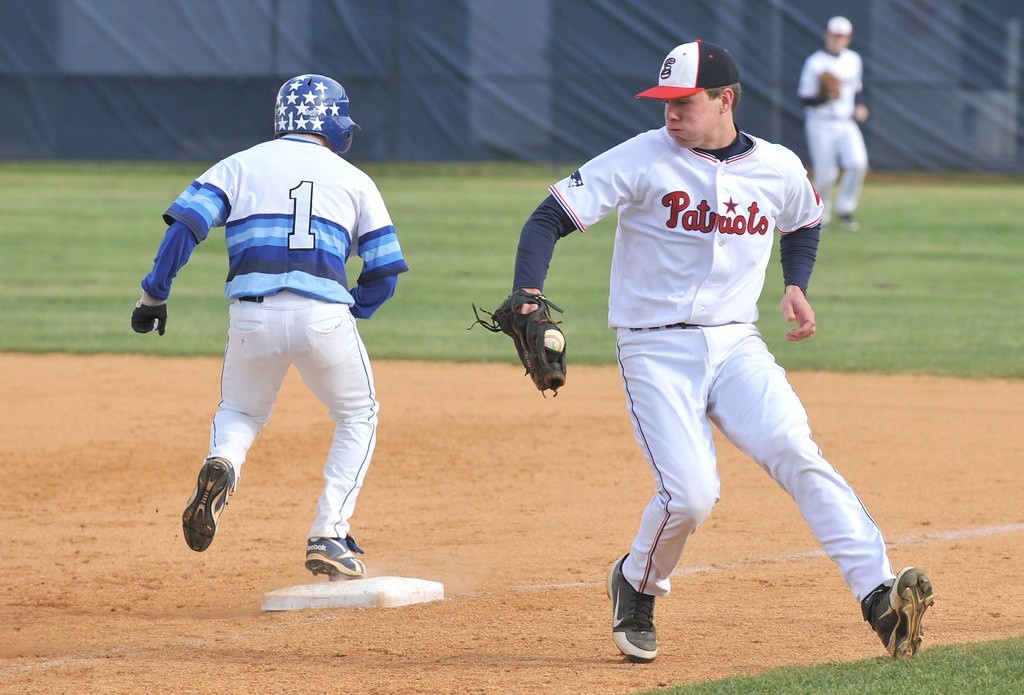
[181,457,236,553]
[605,552,659,663]
[305,534,366,581]
[869,567,935,659]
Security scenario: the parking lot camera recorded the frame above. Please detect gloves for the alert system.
[131,290,168,336]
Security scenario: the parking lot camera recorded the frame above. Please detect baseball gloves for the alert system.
[492,288,571,395]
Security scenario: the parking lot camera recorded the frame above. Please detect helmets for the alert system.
[273,74,360,154]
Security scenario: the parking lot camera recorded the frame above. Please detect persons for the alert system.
[796,16,869,235]
[127,74,409,580]
[492,39,940,666]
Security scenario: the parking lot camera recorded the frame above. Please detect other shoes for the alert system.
[836,213,861,232]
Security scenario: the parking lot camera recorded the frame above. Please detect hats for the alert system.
[635,40,740,99]
[827,15,852,35]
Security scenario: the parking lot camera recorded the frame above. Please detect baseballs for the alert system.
[543,328,566,353]
